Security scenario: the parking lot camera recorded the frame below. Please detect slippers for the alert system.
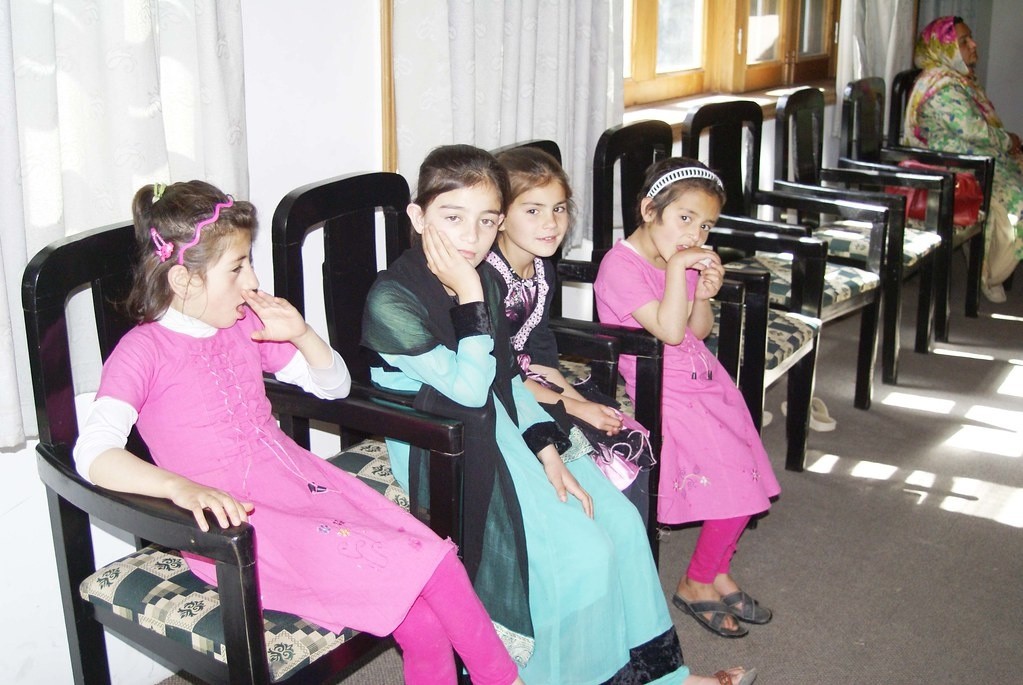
[672,592,749,638]
[780,397,837,432]
[719,591,772,624]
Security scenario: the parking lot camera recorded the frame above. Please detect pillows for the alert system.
[806,211,946,269]
[705,297,814,369]
[77,424,408,681]
[717,243,882,311]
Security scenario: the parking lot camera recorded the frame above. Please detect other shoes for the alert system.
[714,665,758,685]
[981,283,1007,304]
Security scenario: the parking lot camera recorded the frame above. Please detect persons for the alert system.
[483,144,655,527]
[594,156,780,637]
[357,144,758,684]
[902,16,1023,302]
[73,179,526,684]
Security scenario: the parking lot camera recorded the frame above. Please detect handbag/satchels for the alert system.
[885,159,985,226]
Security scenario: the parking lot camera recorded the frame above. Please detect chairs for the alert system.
[17,67,997,685]
